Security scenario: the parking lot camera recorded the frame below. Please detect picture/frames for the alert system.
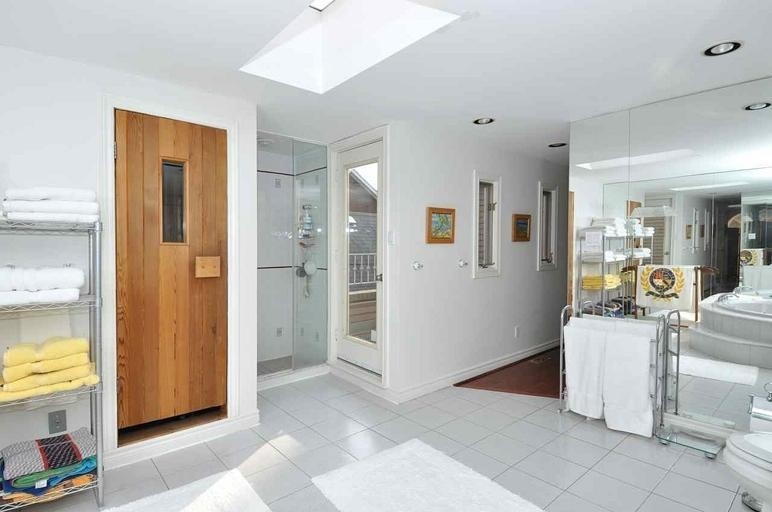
[686,225,692,239]
[426,207,455,244]
[512,214,531,241]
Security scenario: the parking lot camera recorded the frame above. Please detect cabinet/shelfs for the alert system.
[0,220,103,512]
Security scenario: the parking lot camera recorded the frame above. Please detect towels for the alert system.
[0,337,100,403]
[741,248,766,270]
[583,313,675,399]
[0,427,97,504]
[564,316,663,438]
[0,265,84,307]
[636,266,693,311]
[2,189,98,224]
[577,217,655,318]
[627,218,655,315]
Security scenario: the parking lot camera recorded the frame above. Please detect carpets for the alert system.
[103,468,272,512]
[311,438,545,512]
[672,354,759,386]
